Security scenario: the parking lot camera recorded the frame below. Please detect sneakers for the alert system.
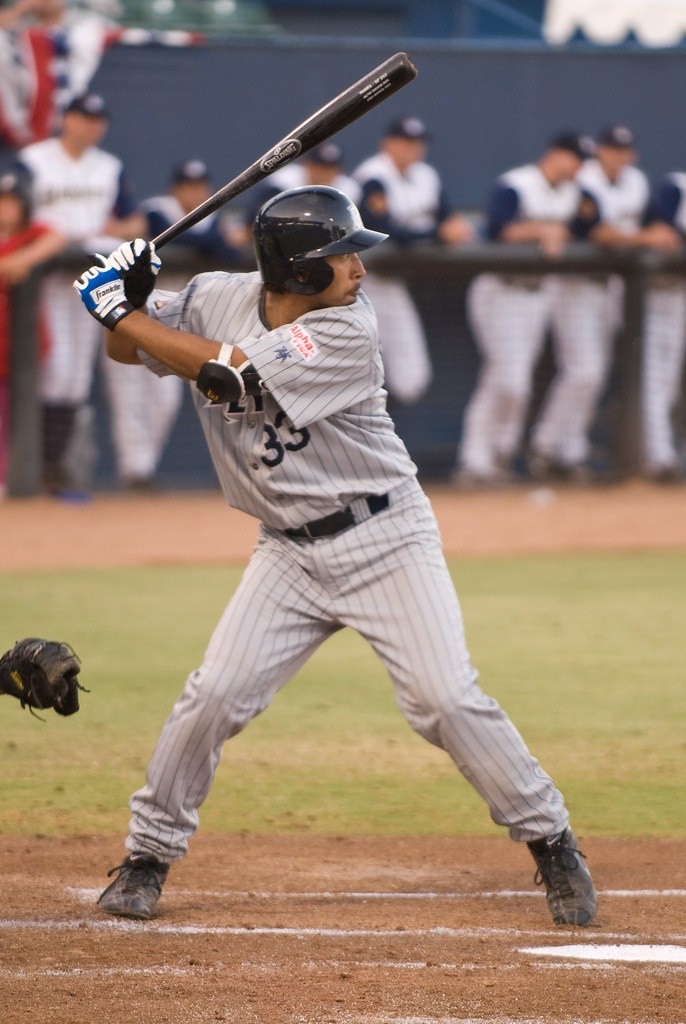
[97,851,170,919]
[527,827,598,929]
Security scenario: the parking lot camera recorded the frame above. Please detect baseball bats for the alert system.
[150,51,419,250]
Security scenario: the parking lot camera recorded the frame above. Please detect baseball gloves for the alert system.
[0,636,94,724]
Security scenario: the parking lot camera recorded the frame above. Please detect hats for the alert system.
[602,128,637,147]
[386,117,427,138]
[176,158,211,183]
[554,132,595,159]
[64,96,112,121]
[310,143,344,164]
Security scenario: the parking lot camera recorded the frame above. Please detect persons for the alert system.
[454,126,686,480]
[73,186,595,925]
[2,0,67,28]
[0,639,80,716]
[352,118,468,398]
[247,141,362,233]
[1,93,241,500]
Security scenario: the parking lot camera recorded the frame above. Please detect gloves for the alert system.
[74,252,136,331]
[107,238,162,309]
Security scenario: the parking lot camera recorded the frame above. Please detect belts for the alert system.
[284,494,389,538]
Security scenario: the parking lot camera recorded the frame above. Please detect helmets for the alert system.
[253,185,390,296]
